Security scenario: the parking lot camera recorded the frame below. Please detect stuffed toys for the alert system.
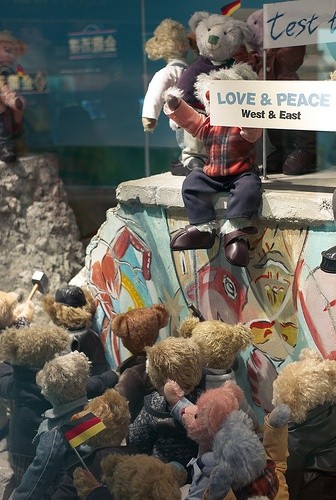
[0,284,336,500]
[142,1,318,267]
[0,39,29,162]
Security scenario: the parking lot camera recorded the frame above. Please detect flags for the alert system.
[60,411,106,448]
